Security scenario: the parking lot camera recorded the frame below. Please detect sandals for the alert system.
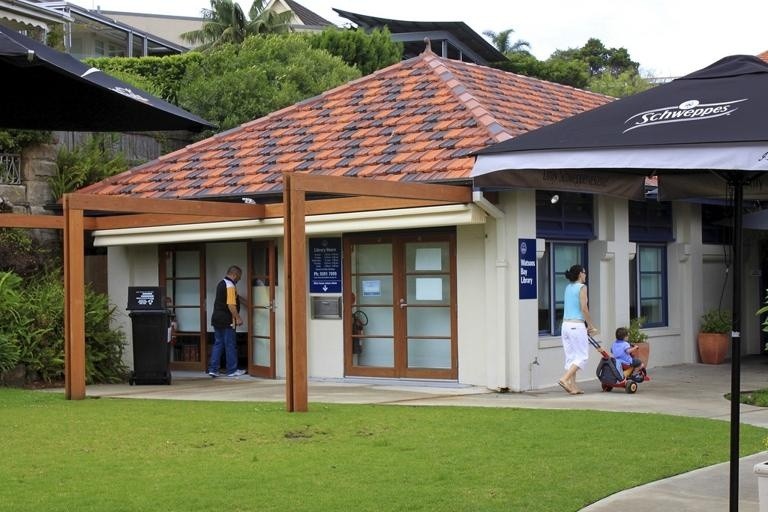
[631,373,642,381]
[558,379,584,394]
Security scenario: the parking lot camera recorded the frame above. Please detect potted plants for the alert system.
[698,311,731,364]
[623,315,652,373]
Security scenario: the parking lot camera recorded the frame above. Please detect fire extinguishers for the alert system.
[352,311,368,354]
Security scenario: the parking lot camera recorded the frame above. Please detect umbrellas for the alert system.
[0,23,223,132]
[468,54,768,512]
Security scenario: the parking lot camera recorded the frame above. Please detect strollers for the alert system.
[588,329,650,391]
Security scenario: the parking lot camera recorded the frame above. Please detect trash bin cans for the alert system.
[126,286,176,385]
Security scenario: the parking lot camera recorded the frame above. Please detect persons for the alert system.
[610,327,642,367]
[208,265,247,378]
[557,265,599,395]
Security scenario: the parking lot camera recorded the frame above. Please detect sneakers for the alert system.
[228,369,247,377]
[209,370,220,376]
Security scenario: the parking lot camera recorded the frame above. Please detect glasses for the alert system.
[582,272,587,274]
